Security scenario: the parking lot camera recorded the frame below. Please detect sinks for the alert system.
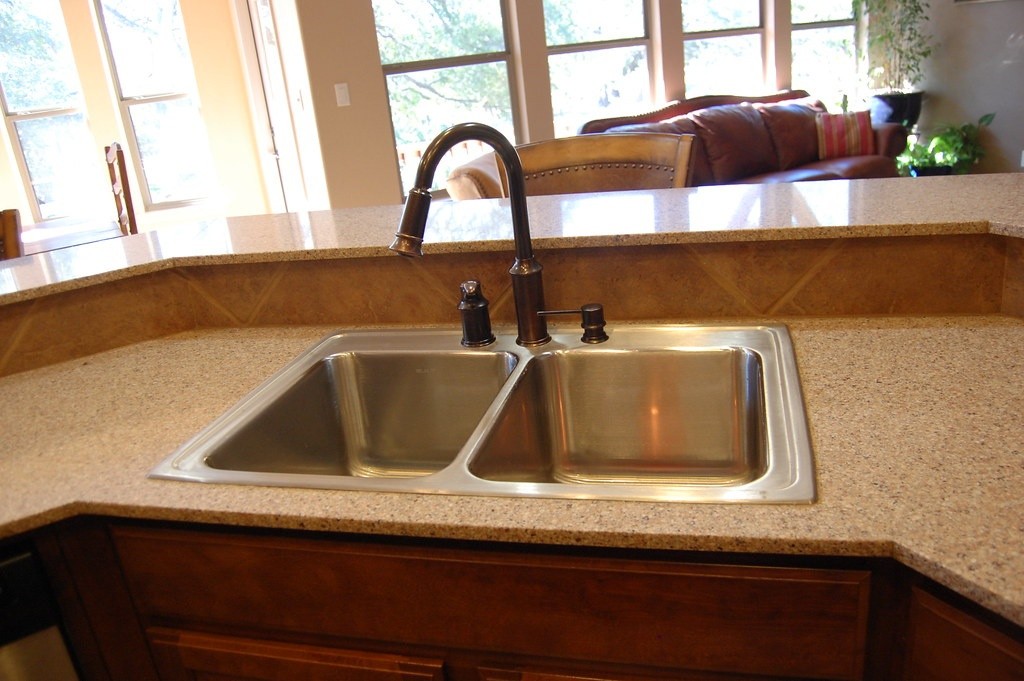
[146,328,524,498]
[459,321,817,506]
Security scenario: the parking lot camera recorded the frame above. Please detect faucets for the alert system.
[388,121,554,348]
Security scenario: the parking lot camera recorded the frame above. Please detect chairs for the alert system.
[443,131,697,200]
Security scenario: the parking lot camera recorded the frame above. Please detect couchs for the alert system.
[576,90,906,185]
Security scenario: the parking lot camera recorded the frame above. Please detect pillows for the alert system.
[814,111,874,161]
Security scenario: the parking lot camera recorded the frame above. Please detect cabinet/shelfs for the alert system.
[0,519,1024,681]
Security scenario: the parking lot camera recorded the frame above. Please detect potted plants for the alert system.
[899,113,1000,178]
[845,0,941,127]
